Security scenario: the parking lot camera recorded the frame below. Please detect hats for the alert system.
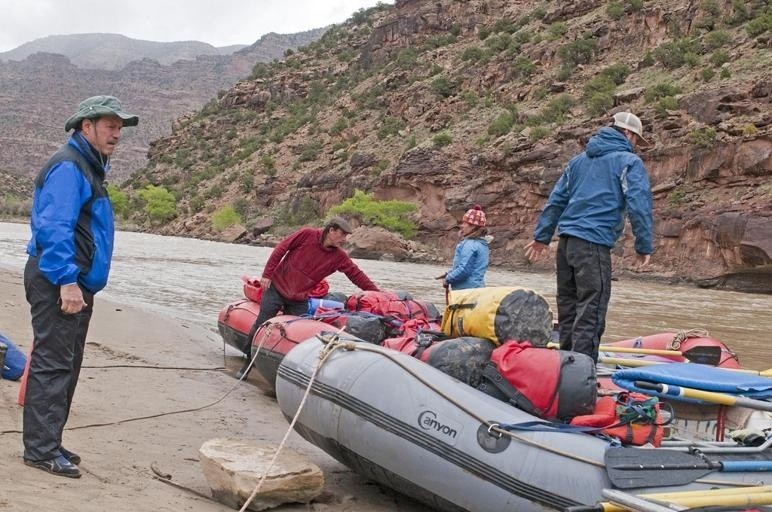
[607,111,649,147]
[62,95,140,132]
[327,217,352,235]
[462,204,487,228]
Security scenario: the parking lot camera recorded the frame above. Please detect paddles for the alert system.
[604,448,772,489]
[546,342,721,364]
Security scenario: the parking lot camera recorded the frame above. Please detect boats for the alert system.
[218,297,347,362]
[248,314,381,393]
[273,329,770,511]
[598,329,742,373]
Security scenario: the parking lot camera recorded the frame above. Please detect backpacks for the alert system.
[239,273,499,395]
[480,339,679,450]
[1,333,27,381]
[441,286,553,348]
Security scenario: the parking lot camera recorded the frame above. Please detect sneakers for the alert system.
[236,352,252,379]
[23,444,83,480]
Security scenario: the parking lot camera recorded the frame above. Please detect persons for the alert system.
[233,216,382,383]
[18,91,140,485]
[443,203,493,293]
[522,109,657,362]
